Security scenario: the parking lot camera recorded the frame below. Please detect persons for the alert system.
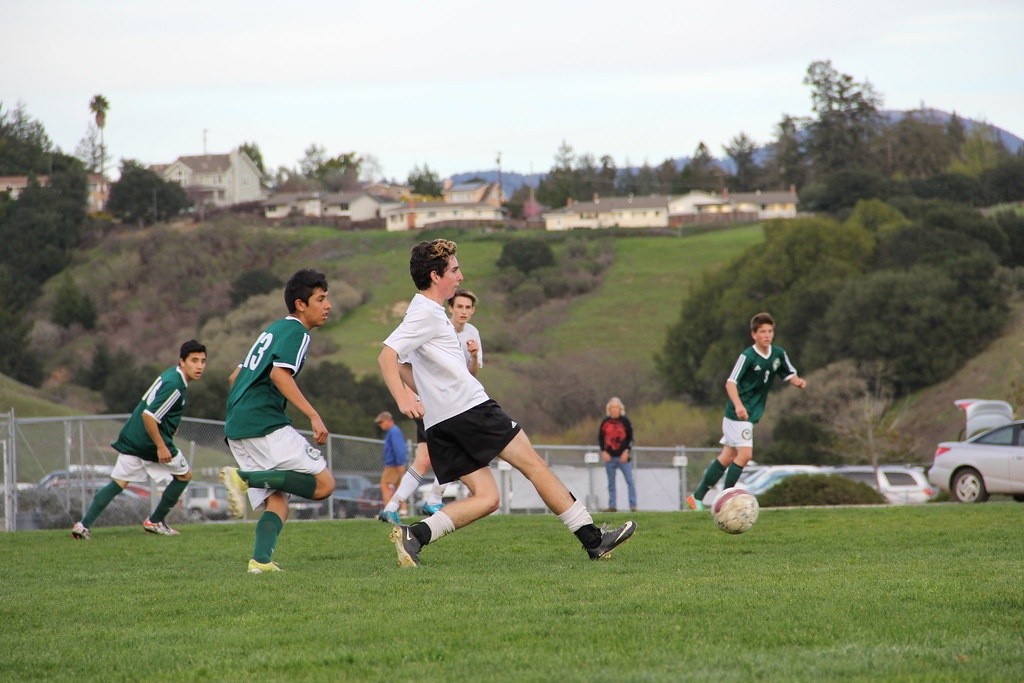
[219,268,336,573]
[685,313,807,511]
[73,340,207,539]
[377,238,637,567]
[374,411,408,520]
[598,397,636,512]
[378,289,483,526]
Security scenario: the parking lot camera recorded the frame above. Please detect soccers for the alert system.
[711,487,759,535]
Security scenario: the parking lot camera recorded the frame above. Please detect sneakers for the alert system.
[581,520,637,561]
[378,509,400,525]
[72,522,90,539]
[143,516,181,536]
[391,524,421,568]
[422,501,444,516]
[220,466,249,519]
[247,559,282,573]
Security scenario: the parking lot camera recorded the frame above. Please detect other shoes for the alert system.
[602,507,616,512]
[685,493,703,511]
[631,508,637,513]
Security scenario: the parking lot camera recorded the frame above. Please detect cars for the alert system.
[923,397,1024,504]
[701,459,939,508]
[0,462,474,530]
